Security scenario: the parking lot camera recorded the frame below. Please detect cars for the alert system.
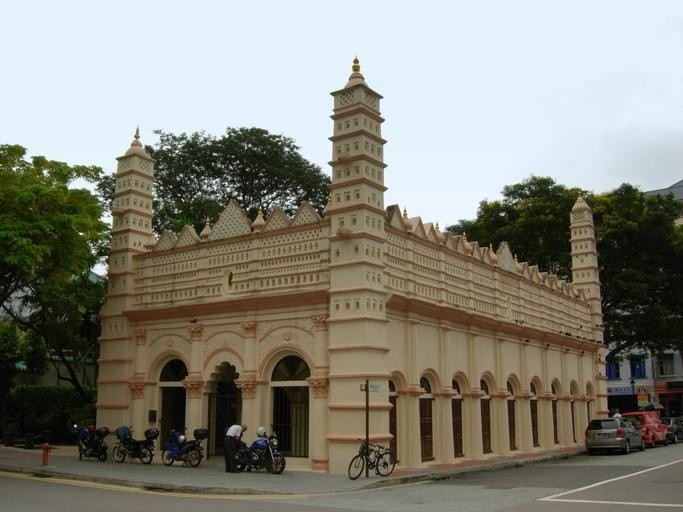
[585,418,645,454]
[662,417,683,444]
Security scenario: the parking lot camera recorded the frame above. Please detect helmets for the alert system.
[88,424,96,433]
[177,434,187,445]
[257,426,266,437]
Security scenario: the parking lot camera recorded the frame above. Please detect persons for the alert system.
[224,424,247,473]
[607,408,642,429]
[79,424,97,456]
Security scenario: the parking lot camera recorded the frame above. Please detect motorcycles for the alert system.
[112,426,158,464]
[233,433,286,474]
[72,422,108,461]
[160,428,210,468]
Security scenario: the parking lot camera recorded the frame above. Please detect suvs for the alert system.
[621,410,673,448]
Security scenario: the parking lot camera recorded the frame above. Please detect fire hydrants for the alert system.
[42,442,53,466]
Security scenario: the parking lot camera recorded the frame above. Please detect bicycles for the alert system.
[347,436,396,480]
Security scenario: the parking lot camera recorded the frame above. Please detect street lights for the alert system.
[630,379,636,397]
